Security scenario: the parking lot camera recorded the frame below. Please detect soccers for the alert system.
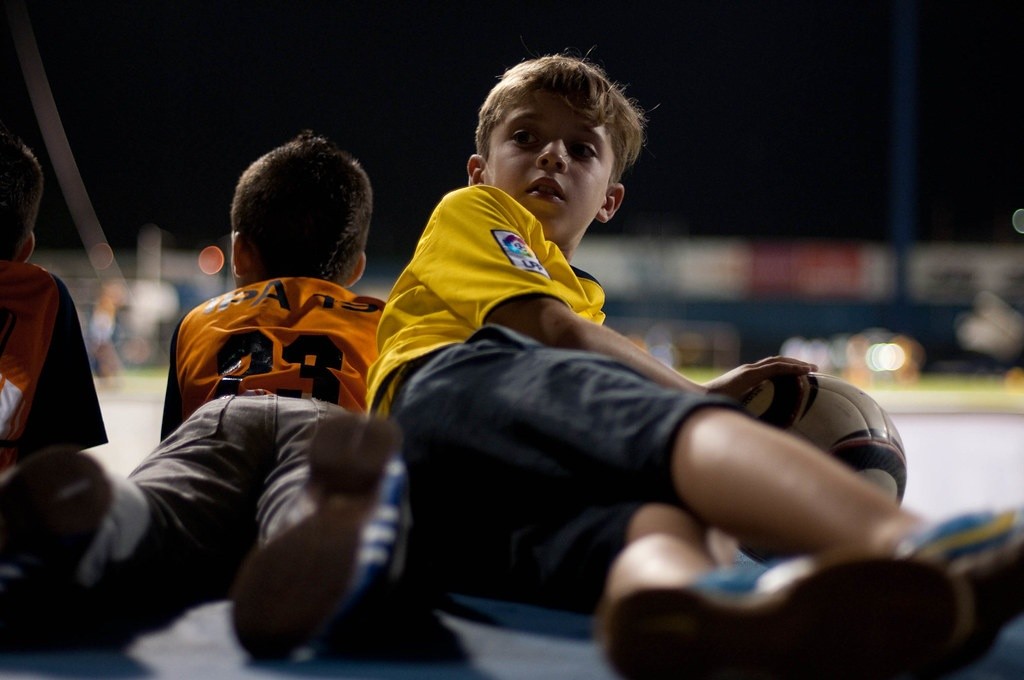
[732,370,907,562]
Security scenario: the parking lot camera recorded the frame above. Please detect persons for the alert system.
[0,134,107,473]
[0,126,409,664]
[363,55,1024,680]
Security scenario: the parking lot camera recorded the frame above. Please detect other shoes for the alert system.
[230,414,396,665]
[899,507,1024,664]
[602,555,971,680]
[0,444,112,642]
